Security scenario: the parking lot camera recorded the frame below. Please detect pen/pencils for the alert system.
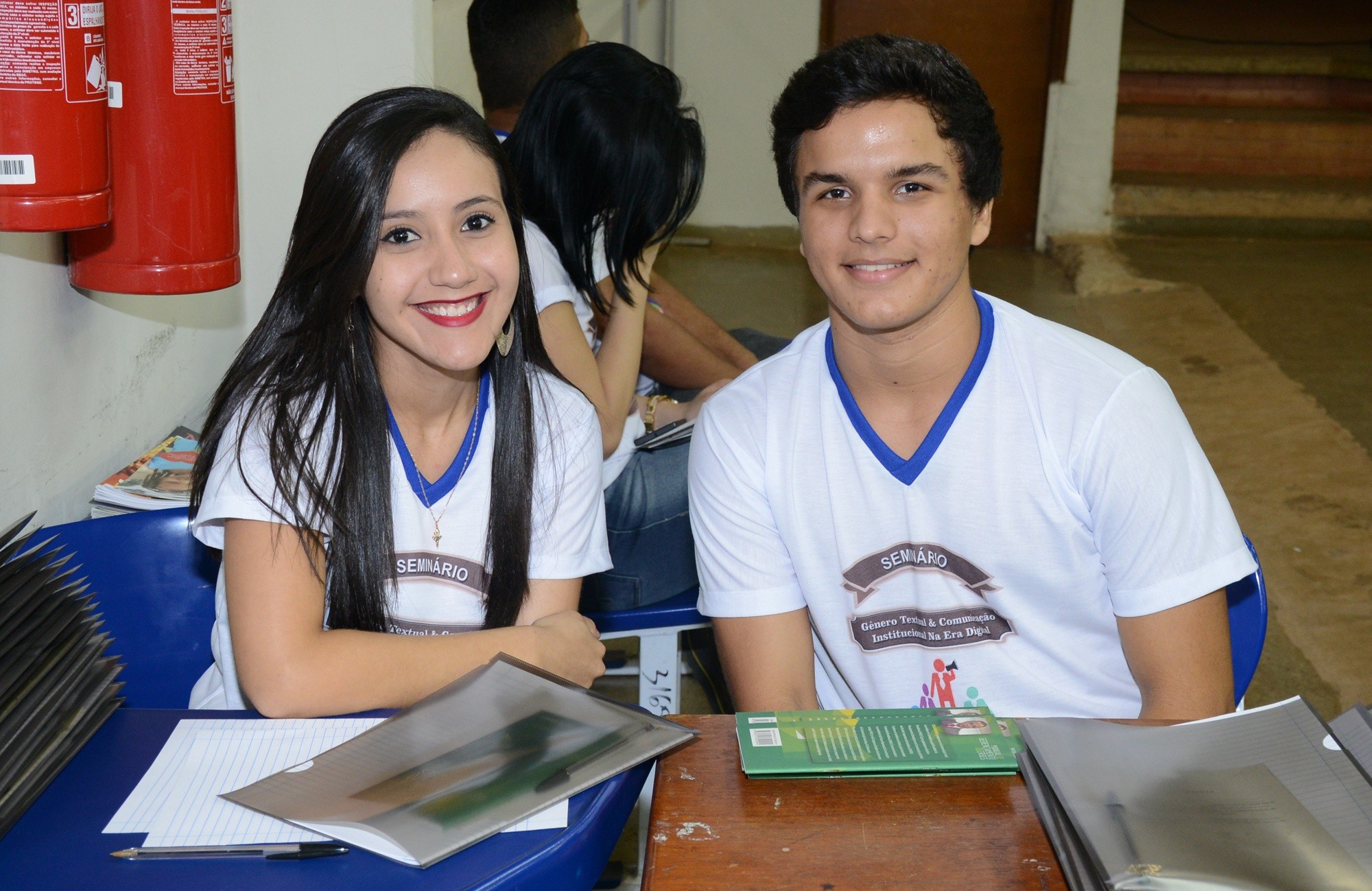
[535,724,655,792]
[108,844,350,860]
[1107,789,1150,884]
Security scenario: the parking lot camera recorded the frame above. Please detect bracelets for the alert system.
[643,393,679,433]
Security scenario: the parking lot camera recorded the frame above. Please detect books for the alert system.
[349,708,628,830]
[86,424,203,521]
[1105,760,1372,891]
[734,704,1030,779]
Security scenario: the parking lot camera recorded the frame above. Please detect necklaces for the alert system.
[396,369,481,548]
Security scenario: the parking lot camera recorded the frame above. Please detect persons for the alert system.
[683,31,1263,731]
[457,0,792,406]
[495,36,738,614]
[185,88,617,703]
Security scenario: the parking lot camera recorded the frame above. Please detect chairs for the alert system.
[22,500,231,711]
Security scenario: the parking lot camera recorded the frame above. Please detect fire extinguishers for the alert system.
[67,0,241,295]
[1,0,113,233]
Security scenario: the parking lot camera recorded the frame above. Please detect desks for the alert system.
[1,700,659,890]
[638,699,1370,891]
